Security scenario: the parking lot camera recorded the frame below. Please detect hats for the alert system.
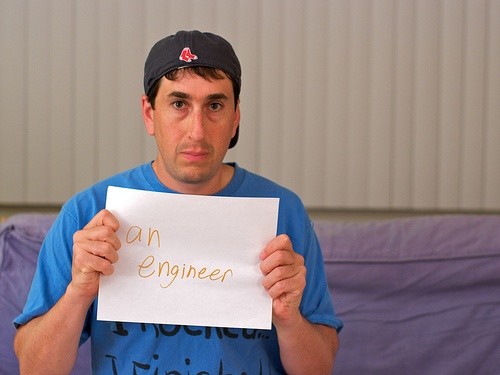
[144,30,241,149]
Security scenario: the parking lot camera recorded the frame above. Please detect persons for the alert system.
[13,30,339,375]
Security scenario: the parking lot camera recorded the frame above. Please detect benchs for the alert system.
[0,213,499,374]
